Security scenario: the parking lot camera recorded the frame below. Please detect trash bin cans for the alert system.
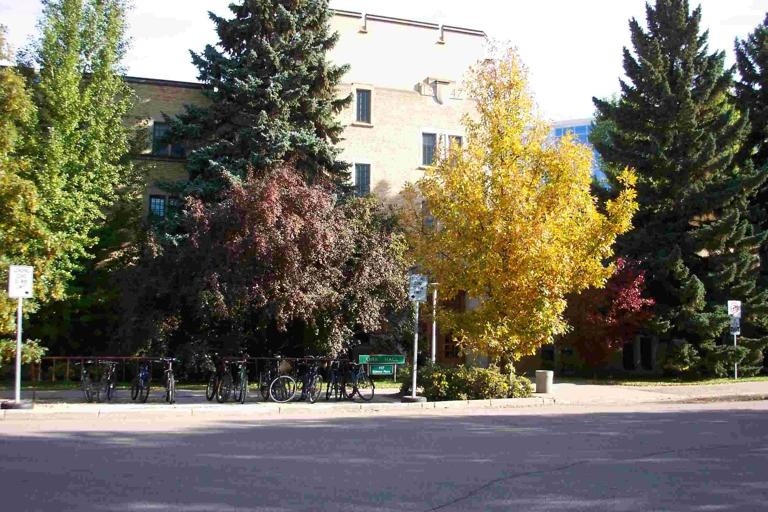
[535,369,554,393]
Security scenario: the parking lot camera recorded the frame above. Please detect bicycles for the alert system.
[206,351,375,403]
[74,357,177,404]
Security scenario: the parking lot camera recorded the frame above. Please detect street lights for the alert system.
[429,282,439,364]
